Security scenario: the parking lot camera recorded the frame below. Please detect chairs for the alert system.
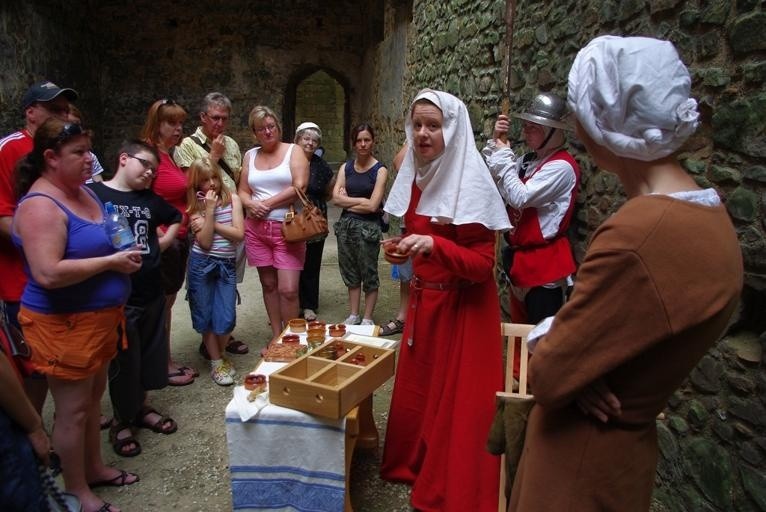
[494,321,536,511]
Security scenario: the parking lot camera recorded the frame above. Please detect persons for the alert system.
[505,36,742,512]
[14,117,141,512]
[290,122,334,321]
[138,99,200,388]
[85,140,184,457]
[0,81,78,455]
[328,124,387,324]
[379,88,521,512]
[237,106,330,358]
[0,352,84,511]
[482,93,579,389]
[66,104,113,430]
[379,139,415,336]
[185,157,244,385]
[173,93,249,360]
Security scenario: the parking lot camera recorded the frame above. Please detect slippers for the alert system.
[181,366,200,377]
[168,369,194,386]
[87,469,139,489]
[97,501,121,512]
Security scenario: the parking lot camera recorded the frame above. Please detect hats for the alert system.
[23,79,79,110]
[295,121,321,134]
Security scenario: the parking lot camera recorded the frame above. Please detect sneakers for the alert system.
[221,352,236,376]
[359,319,375,325]
[210,358,234,385]
[344,314,360,325]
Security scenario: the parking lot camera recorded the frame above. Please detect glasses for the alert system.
[254,123,275,131]
[51,122,81,142]
[157,97,175,110]
[126,153,158,176]
[204,111,227,121]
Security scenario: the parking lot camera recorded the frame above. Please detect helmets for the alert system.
[512,93,573,132]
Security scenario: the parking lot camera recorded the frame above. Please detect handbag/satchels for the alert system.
[281,183,329,244]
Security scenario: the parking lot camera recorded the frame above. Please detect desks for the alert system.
[224,320,380,512]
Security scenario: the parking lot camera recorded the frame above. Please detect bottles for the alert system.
[106,202,139,254]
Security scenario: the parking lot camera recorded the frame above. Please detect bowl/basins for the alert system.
[306,330,326,337]
[384,246,412,264]
[281,334,300,346]
[244,374,267,391]
[287,318,307,333]
[307,337,325,346]
[319,350,335,359]
[328,323,346,338]
[308,321,326,329]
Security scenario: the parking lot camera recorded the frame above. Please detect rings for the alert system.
[414,245,419,249]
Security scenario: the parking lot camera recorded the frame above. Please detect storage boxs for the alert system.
[267,337,397,422]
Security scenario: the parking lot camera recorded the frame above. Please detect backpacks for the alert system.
[345,159,390,233]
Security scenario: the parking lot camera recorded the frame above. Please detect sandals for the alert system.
[109,422,141,457]
[225,336,248,354]
[303,308,317,321]
[133,406,177,434]
[379,318,405,336]
